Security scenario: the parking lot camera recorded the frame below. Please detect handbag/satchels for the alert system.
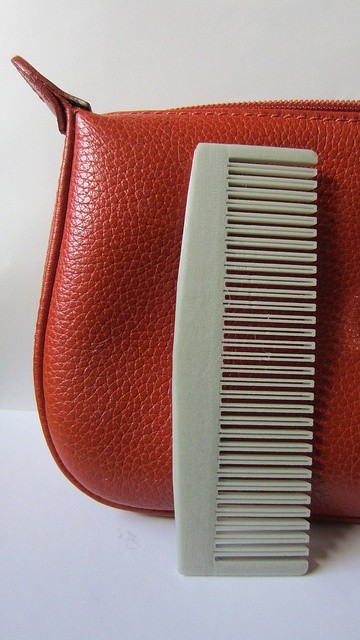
[13,56,359,522]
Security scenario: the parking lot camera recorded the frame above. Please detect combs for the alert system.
[171,141,322,577]
